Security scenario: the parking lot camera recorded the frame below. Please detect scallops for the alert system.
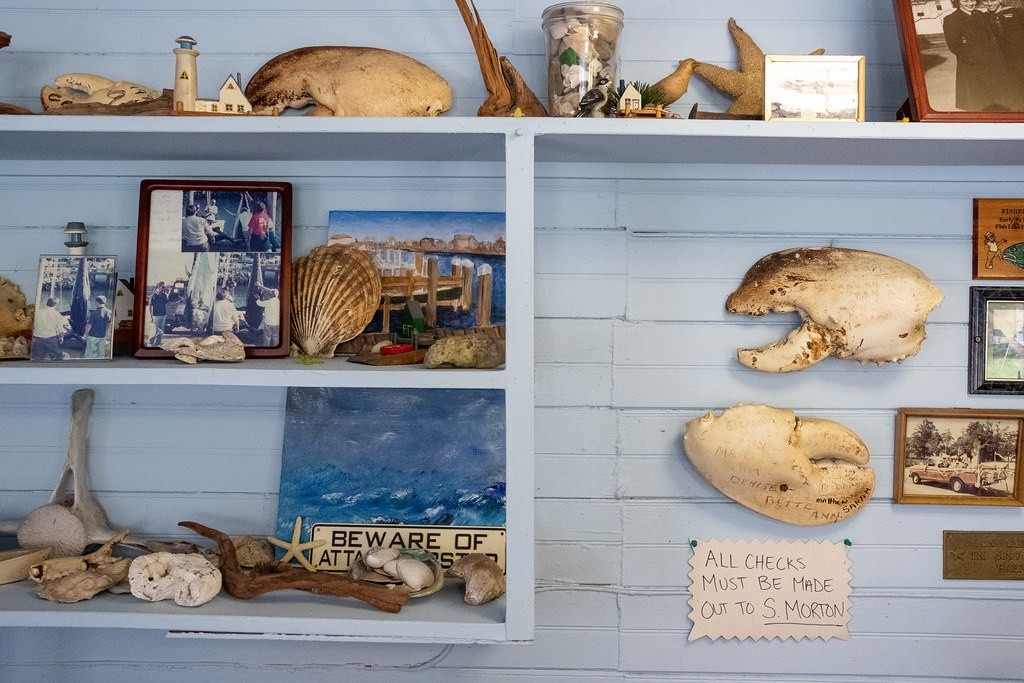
[290,243,382,359]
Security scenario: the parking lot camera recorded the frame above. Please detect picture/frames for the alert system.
[968,286,1024,395]
[893,408,1024,507]
[893,0,1024,123]
[132,178,293,356]
[764,54,866,122]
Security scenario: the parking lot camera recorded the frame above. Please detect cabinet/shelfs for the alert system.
[0,116,1024,642]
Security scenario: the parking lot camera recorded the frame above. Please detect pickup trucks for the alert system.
[907,457,1002,493]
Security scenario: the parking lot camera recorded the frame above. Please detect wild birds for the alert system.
[646,58,701,117]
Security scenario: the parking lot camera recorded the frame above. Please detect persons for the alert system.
[182,199,219,253]
[81,295,111,359]
[147,282,169,347]
[204,281,241,335]
[252,281,280,347]
[31,296,65,362]
[232,197,281,253]
[942,0,1024,113]
